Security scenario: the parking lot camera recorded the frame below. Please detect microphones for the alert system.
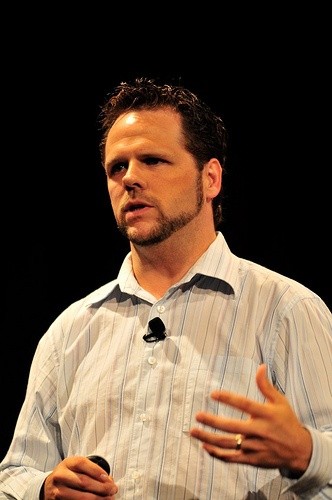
[149,317,166,340]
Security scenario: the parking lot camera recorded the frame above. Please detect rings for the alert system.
[235,435,243,449]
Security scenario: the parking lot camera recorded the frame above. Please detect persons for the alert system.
[1,78,331,500]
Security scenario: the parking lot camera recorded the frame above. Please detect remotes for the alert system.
[87,456,111,476]
